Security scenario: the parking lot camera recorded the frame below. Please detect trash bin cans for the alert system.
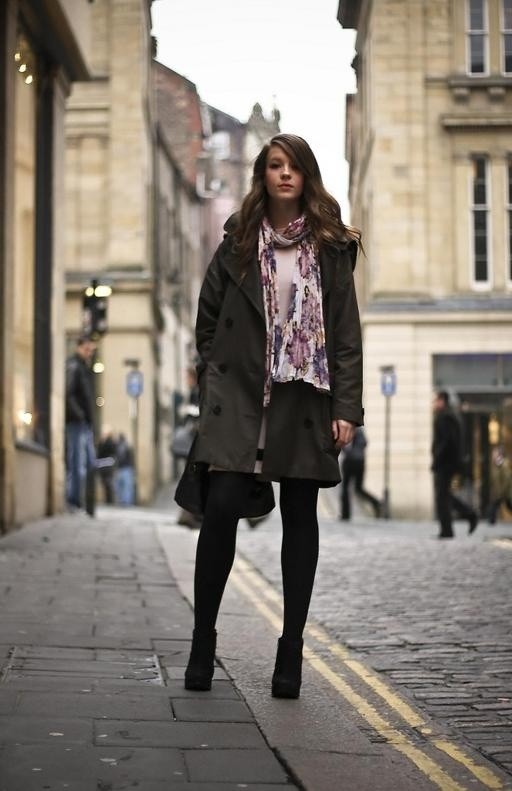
[114,467,137,505]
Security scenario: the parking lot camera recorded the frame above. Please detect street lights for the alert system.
[80,277,110,515]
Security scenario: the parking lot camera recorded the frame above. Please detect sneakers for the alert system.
[339,497,382,523]
[436,513,479,540]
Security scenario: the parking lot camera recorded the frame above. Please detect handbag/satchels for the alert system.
[172,424,278,522]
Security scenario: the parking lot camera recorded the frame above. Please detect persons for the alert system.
[66,334,99,510]
[93,426,112,505]
[175,361,271,531]
[426,388,512,539]
[185,132,366,700]
[339,421,381,519]
[112,429,133,504]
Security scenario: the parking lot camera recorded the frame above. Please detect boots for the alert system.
[182,627,220,691]
[268,635,306,700]
